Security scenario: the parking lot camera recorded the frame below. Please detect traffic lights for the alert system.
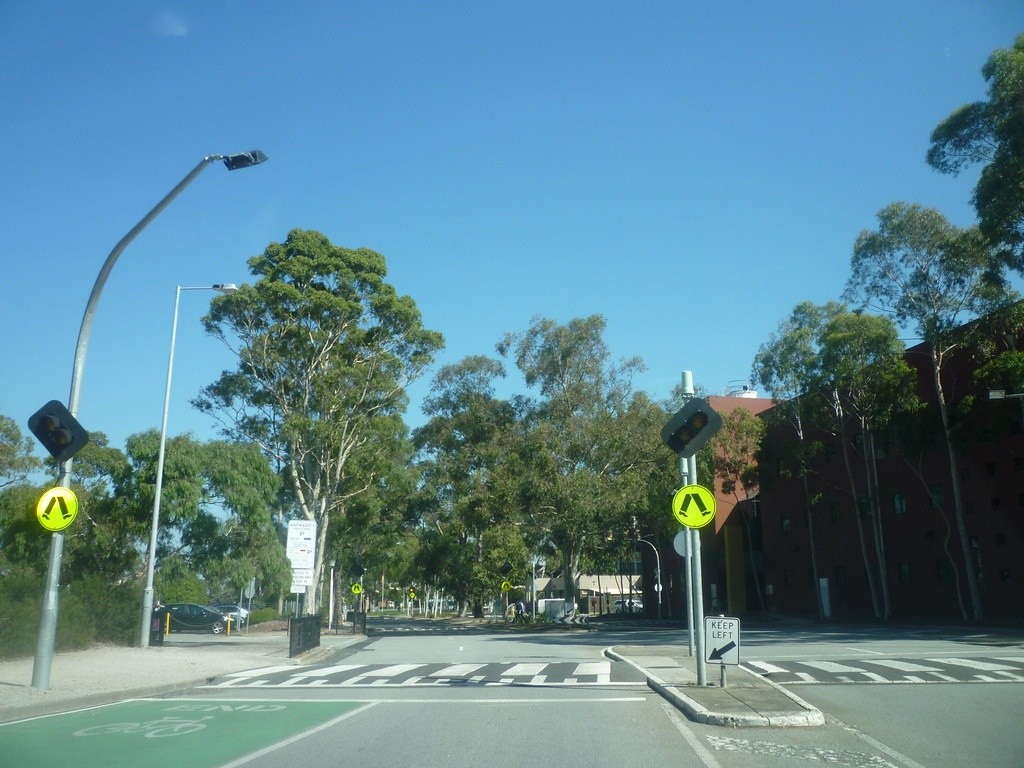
[28,399,91,463]
[499,561,514,575]
[351,563,365,578]
[659,397,723,459]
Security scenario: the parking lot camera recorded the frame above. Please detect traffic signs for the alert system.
[703,615,741,665]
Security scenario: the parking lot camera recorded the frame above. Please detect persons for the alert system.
[512,598,525,624]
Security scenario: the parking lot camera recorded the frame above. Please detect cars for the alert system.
[154,601,245,635]
[204,602,249,627]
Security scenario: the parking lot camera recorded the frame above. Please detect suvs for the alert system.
[613,598,643,615]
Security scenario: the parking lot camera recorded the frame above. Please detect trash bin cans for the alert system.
[149,605,166,645]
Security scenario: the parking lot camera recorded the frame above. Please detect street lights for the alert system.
[27,144,272,689]
[514,522,536,623]
[623,537,662,622]
[357,521,409,613]
[138,282,240,648]
[326,560,337,632]
